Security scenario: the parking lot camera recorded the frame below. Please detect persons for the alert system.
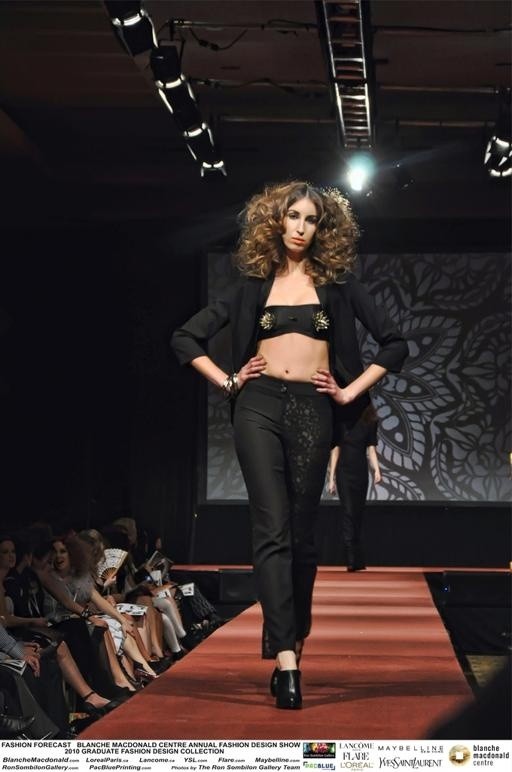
[172,181,409,706]
[326,391,381,571]
[1,517,226,739]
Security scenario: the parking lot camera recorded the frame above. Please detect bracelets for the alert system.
[221,372,239,401]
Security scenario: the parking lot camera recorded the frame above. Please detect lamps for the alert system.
[104,0,159,59]
[149,17,233,178]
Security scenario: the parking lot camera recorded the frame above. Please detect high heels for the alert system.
[79,667,161,714]
[0,713,37,740]
[275,668,303,710]
[270,669,278,698]
